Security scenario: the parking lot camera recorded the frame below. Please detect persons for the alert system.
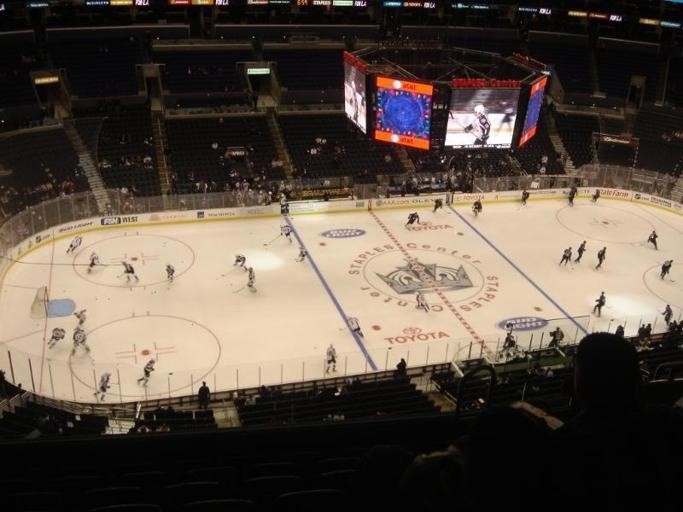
[559,246,573,265]
[593,247,607,270]
[574,240,588,264]
[280,224,293,243]
[244,266,257,293]
[71,326,89,356]
[70,307,88,325]
[0,369,22,402]
[136,358,155,389]
[346,315,364,338]
[324,344,337,373]
[416,291,430,313]
[134,403,174,433]
[232,255,245,267]
[121,262,138,283]
[87,253,99,272]
[234,382,381,397]
[165,264,175,283]
[648,230,658,250]
[2,41,680,226]
[196,381,211,410]
[661,304,671,323]
[589,291,606,317]
[92,371,112,401]
[395,358,406,376]
[63,236,83,253]
[660,259,674,281]
[45,327,64,350]
[492,317,681,348]
[404,212,420,227]
[294,247,308,264]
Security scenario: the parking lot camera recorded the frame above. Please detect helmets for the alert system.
[474,104,483,112]
[351,81,355,91]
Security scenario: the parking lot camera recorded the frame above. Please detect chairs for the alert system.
[0,336,683,512]
